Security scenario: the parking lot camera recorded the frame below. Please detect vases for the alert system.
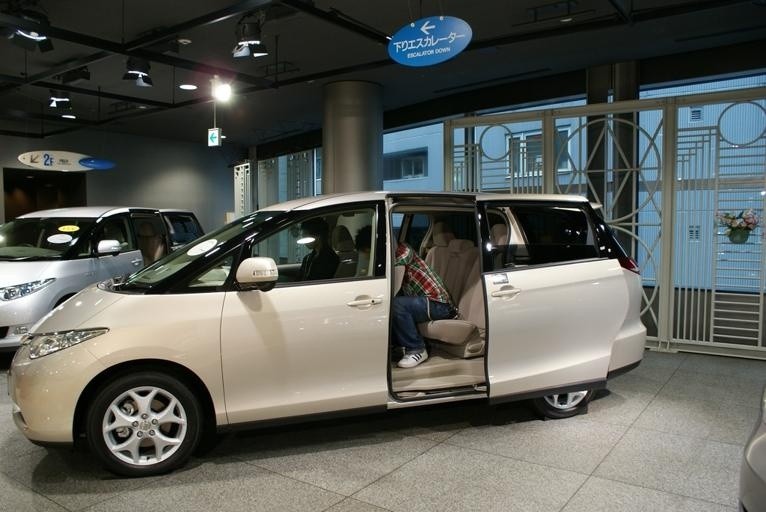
[729,229,750,244]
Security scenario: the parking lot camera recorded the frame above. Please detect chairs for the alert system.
[105,227,127,251]
[417,222,507,345]
[136,221,165,267]
[331,226,359,278]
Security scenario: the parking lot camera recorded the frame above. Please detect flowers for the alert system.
[714,208,766,238]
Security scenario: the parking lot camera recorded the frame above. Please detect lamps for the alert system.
[1,6,270,120]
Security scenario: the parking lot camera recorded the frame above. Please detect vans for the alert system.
[0,205,207,354]
[6,185,650,474]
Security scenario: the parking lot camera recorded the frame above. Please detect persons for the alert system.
[352,223,459,370]
[289,216,341,282]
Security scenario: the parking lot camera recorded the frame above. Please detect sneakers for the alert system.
[397,348,428,368]
